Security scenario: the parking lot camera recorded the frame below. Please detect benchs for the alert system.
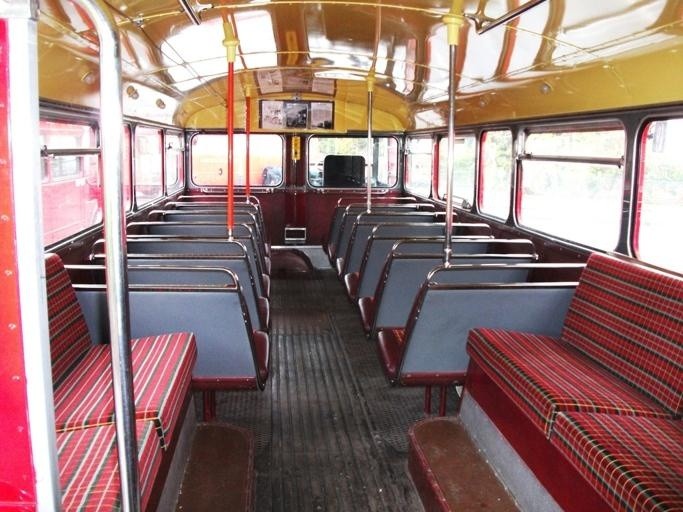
[465,251,682,439]
[44,253,196,451]
[53,420,161,512]
[549,412,682,512]
